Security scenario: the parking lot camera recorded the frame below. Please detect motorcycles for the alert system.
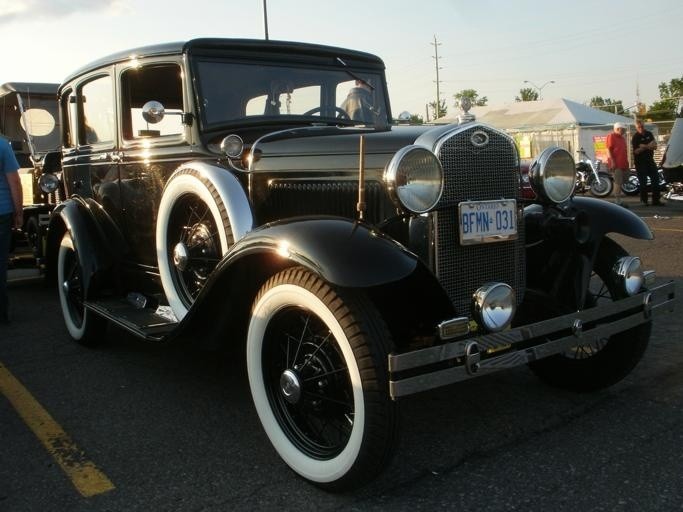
[513,146,613,200]
[619,151,683,202]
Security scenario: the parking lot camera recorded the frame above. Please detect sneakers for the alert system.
[617,200,628,206]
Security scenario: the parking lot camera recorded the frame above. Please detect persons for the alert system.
[606,122,630,209]
[337,77,375,125]
[0,134,25,322]
[631,119,668,207]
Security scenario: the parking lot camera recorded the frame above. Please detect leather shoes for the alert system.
[641,200,649,206]
[652,200,665,207]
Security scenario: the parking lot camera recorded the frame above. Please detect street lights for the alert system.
[522,79,556,104]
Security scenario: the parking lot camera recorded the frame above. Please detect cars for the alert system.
[42,39,672,487]
[1,81,75,288]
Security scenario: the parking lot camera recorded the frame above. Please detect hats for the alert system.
[613,122,627,129]
